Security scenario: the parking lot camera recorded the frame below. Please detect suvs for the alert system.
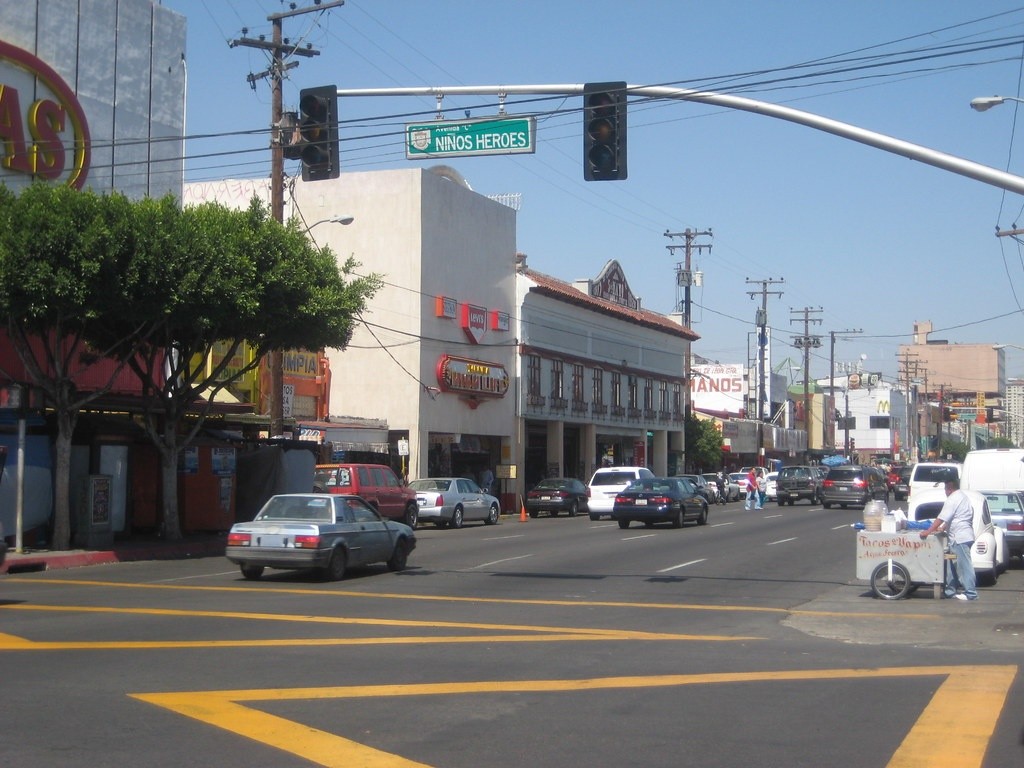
[739,466,769,479]
[312,464,419,529]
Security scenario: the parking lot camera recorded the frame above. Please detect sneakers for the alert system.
[956,593,980,600]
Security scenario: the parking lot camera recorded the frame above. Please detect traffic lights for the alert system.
[987,409,993,423]
[583,81,628,181]
[298,84,340,182]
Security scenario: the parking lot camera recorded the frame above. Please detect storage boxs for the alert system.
[881,520,901,532]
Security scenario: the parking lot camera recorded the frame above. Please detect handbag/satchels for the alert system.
[746,486,751,492]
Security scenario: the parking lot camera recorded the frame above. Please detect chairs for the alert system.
[660,486,670,491]
[285,505,313,518]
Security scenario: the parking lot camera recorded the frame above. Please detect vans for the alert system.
[908,448,1024,501]
[587,466,656,521]
[776,467,824,506]
[820,465,889,509]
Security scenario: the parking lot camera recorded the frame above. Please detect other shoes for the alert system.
[744,506,751,511]
[755,507,763,510]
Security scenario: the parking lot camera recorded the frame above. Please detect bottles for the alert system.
[864,500,907,533]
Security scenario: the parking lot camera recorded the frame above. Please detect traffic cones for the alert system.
[520,506,528,522]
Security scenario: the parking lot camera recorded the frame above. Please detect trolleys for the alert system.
[856,524,956,600]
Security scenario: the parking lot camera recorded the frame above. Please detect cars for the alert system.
[613,472,740,529]
[905,489,1024,588]
[526,478,589,518]
[406,477,501,528]
[887,465,913,501]
[227,493,416,581]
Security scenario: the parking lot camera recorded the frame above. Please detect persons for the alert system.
[920,479,980,601]
[715,470,729,499]
[756,471,767,509]
[745,467,763,510]
[479,464,493,495]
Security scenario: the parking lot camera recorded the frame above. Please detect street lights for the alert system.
[271,214,355,441]
[758,367,801,466]
[845,394,875,459]
